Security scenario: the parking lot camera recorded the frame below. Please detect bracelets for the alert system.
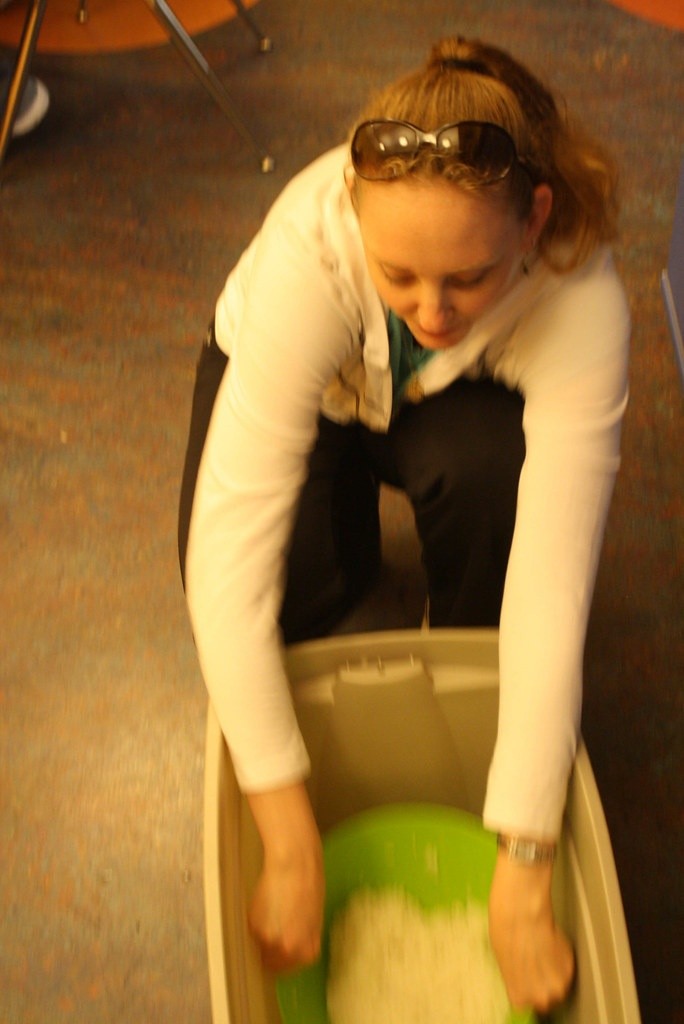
[496,831,557,865]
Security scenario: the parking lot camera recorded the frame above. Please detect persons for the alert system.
[178,31,632,1014]
[1,64,49,137]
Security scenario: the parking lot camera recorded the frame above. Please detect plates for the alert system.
[276,804,534,1024]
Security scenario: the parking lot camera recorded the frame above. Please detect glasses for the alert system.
[351,118,537,188]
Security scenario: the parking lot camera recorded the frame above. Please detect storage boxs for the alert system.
[201,623,641,1024]
